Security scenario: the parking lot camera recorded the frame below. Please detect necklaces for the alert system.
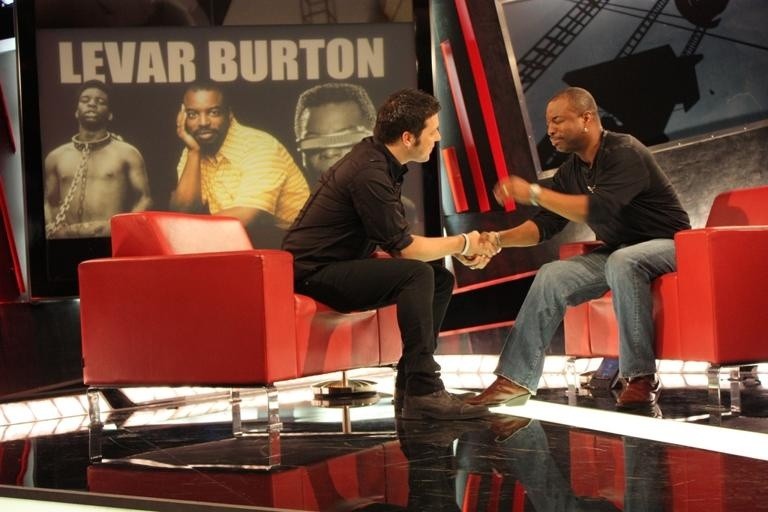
[577,130,606,195]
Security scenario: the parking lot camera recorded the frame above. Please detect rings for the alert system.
[175,122,181,128]
[503,182,509,197]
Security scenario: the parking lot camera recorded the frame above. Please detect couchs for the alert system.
[77,211,404,436]
[560,184,768,410]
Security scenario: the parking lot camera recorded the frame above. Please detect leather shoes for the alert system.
[394,388,475,418]
[458,376,531,405]
[616,374,663,408]
[402,390,491,424]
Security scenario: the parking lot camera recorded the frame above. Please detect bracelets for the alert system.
[459,232,470,257]
[490,230,504,254]
[527,183,541,208]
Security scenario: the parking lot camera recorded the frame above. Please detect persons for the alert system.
[279,87,491,420]
[294,81,418,231]
[42,79,153,240]
[464,86,691,411]
[167,76,313,249]
[397,417,610,512]
